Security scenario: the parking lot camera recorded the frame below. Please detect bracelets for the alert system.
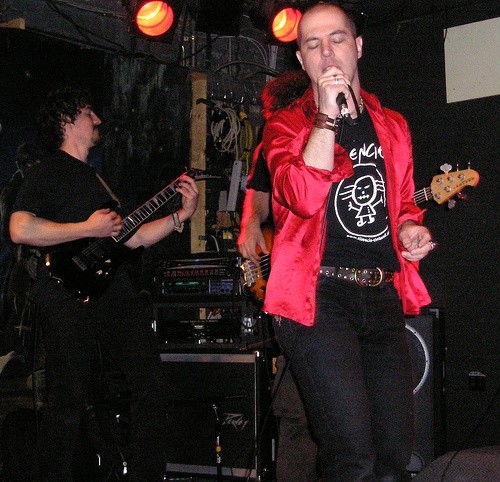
[173,214,184,232]
[314,112,338,132]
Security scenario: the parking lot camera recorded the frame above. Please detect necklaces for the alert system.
[316,96,363,115]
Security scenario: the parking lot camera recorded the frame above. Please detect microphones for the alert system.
[336,92,350,119]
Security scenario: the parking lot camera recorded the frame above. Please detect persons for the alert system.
[9,90,199,482]
[264,3,435,482]
[236,72,319,482]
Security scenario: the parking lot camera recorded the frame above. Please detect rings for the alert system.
[116,220,119,225]
[333,75,337,78]
[336,78,339,83]
[429,242,435,249]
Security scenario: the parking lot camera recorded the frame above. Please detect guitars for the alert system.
[42,167,226,301]
[241,162,481,301]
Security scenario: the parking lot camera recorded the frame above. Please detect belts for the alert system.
[318,266,396,287]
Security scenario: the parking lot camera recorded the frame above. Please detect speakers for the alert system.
[158,343,279,482]
[401,309,447,472]
